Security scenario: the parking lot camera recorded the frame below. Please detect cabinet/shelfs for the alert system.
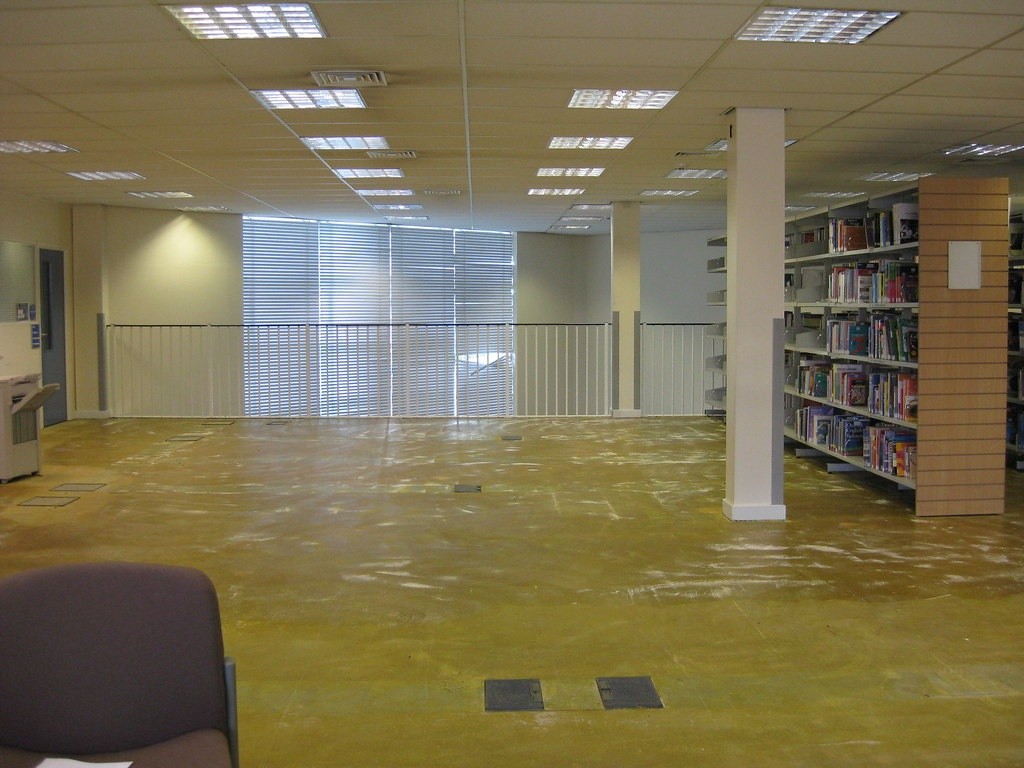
[704,233,727,417]
[784,177,1008,517]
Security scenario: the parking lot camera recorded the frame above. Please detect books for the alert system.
[794,203,919,480]
[1004,216,1024,473]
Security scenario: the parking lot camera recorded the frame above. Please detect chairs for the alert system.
[0,559,238,768]
